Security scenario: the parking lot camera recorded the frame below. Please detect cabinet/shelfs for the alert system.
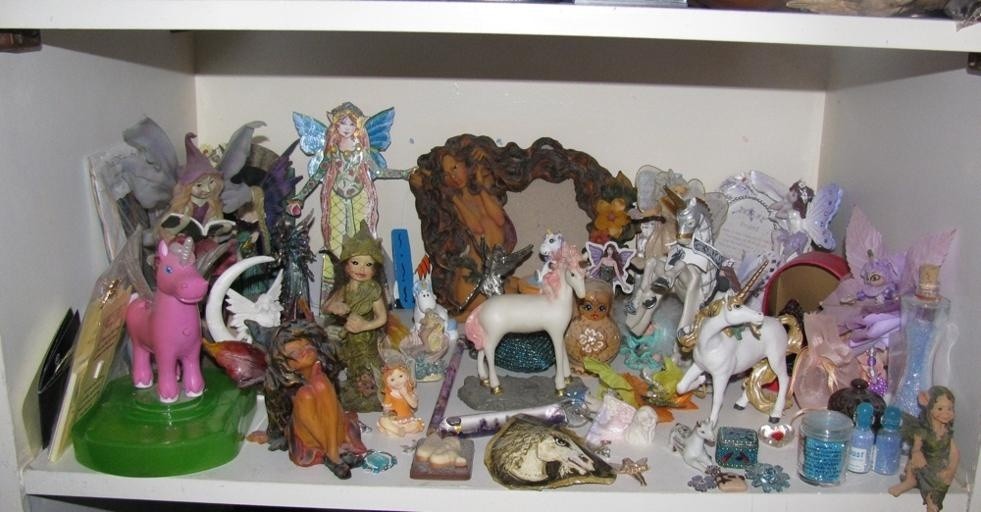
[2,3,981,512]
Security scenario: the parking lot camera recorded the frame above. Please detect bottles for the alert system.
[790,263,954,489]
